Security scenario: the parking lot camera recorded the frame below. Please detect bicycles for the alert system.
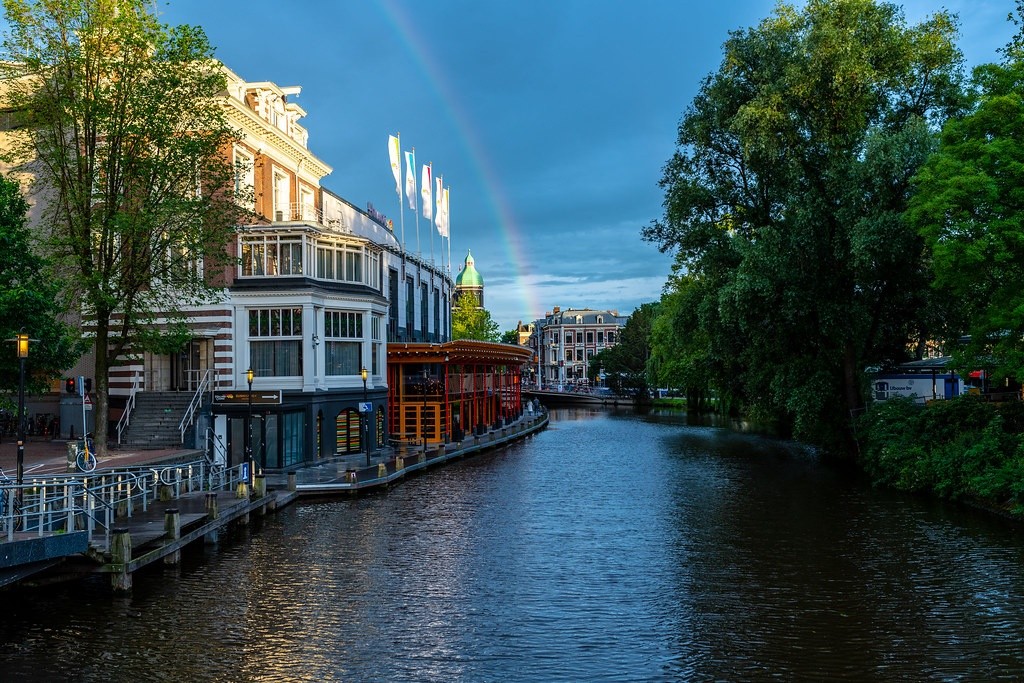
[0,467,22,538]
[77,432,98,473]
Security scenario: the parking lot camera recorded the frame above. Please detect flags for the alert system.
[388,134,400,194]
[442,188,448,237]
[435,177,442,235]
[404,151,416,210]
[421,164,431,220]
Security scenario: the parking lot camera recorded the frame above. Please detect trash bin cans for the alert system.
[477,423,483,435]
[66,441,84,473]
[496,419,502,428]
[452,429,462,442]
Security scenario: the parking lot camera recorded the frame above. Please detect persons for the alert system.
[527,399,533,416]
[487,386,492,395]
[533,397,539,414]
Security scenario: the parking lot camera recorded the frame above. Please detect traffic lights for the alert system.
[66,378,76,393]
[85,378,93,393]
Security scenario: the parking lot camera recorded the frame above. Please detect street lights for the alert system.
[361,365,371,465]
[14,332,29,512]
[240,367,255,490]
